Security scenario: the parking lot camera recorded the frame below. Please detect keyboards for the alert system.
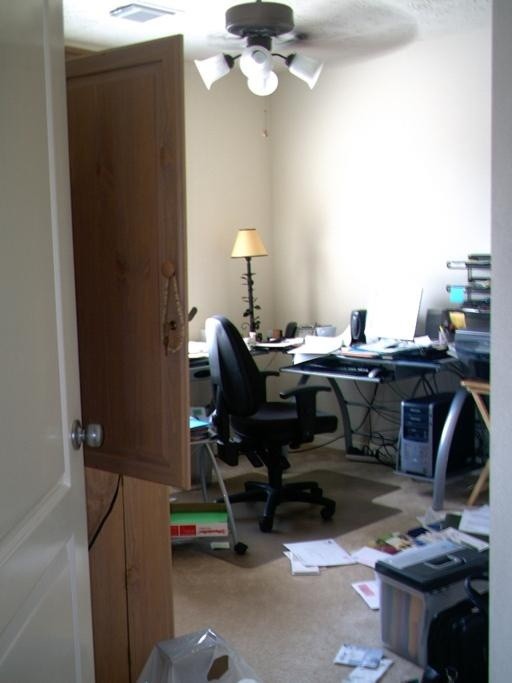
[303,361,368,374]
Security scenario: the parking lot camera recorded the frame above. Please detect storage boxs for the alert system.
[375,533,489,670]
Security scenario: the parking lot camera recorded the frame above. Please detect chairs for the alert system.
[205,315,340,536]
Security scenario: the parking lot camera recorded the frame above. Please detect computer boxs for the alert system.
[402,392,475,476]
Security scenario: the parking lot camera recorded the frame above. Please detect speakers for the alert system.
[350,309,367,343]
[286,322,297,337]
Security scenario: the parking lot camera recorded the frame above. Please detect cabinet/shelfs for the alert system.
[69,39,189,682]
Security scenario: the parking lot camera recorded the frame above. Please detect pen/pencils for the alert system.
[439,325,449,344]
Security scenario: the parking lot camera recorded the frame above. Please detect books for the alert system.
[289,553,320,574]
[189,404,217,441]
[365,513,488,561]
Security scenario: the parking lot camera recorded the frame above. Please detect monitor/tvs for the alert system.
[368,280,424,350]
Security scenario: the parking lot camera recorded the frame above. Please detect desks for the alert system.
[280,352,454,465]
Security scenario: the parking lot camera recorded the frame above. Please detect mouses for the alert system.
[368,367,384,378]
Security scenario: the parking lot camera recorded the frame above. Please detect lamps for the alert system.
[194,0,324,99]
[231,228,269,341]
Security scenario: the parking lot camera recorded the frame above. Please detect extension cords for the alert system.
[346,455,381,463]
